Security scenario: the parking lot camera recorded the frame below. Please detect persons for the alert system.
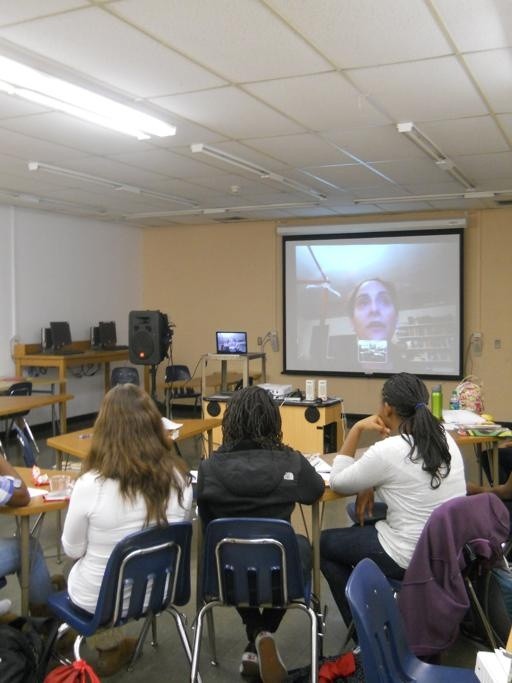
[318,371,467,652]
[331,277,416,374]
[58,382,194,677]
[0,453,66,635]
[195,385,324,683]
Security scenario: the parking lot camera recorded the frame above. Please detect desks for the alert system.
[15,341,149,436]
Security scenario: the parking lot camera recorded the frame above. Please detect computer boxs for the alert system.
[41,328,51,355]
[90,327,98,351]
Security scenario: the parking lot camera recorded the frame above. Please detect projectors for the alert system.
[256,381,292,401]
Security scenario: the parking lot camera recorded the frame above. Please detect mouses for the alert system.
[316,397,322,403]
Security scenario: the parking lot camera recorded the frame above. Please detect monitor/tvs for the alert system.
[49,321,72,354]
[98,320,116,351]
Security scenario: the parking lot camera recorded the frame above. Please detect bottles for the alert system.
[448,390,459,410]
[430,383,443,422]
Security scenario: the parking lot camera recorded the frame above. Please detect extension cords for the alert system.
[284,395,302,401]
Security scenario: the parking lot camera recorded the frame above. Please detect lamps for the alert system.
[395,121,477,193]
[188,142,330,202]
[27,161,202,206]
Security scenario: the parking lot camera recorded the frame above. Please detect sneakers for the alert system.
[255,631,288,683]
[96,637,138,676]
[51,575,66,591]
[240,642,258,676]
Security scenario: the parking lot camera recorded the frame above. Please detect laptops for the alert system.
[207,331,248,357]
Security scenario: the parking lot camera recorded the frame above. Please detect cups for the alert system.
[49,475,71,499]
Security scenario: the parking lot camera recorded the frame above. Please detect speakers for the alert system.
[128,310,171,367]
[318,379,328,401]
[305,379,314,401]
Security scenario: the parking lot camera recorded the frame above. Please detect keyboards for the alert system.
[55,350,84,355]
[104,345,128,352]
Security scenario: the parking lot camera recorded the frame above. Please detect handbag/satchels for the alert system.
[450,375,484,416]
[0,615,59,683]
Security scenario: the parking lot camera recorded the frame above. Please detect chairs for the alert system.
[338,491,510,657]
[345,557,479,682]
[0,365,511,618]
[189,516,323,683]
[36,521,203,683]
